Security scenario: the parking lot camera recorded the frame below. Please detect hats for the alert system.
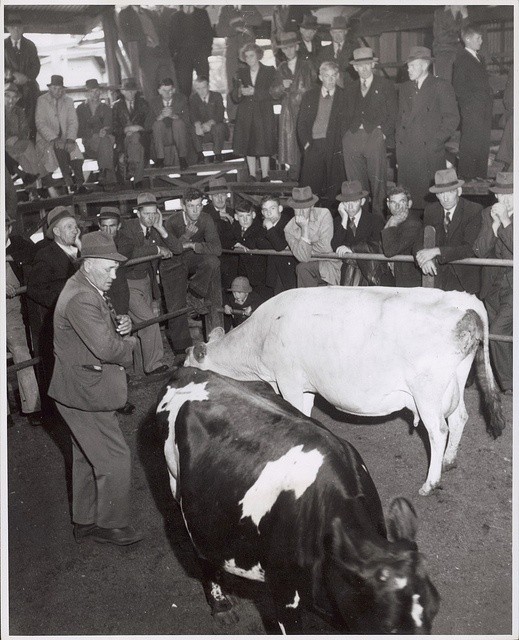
[97,205,121,219]
[297,14,319,27]
[487,171,513,195]
[203,177,232,194]
[335,180,369,201]
[6,12,25,26]
[76,231,128,262]
[275,31,301,48]
[326,17,352,30]
[85,79,103,92]
[115,77,139,90]
[349,47,378,65]
[6,214,16,226]
[48,75,67,87]
[285,186,319,208]
[401,46,436,65]
[132,192,163,209]
[428,168,464,193]
[226,275,253,293]
[46,206,82,239]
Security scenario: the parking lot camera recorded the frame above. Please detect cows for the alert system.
[182,285,508,496]
[154,365,444,635]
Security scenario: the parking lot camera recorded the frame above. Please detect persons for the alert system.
[296,14,322,60]
[451,22,492,181]
[171,6,211,97]
[150,6,178,65]
[316,16,362,71]
[283,185,343,287]
[4,13,40,81]
[85,206,133,315]
[5,82,40,192]
[396,46,461,202]
[270,30,315,182]
[216,5,263,91]
[472,171,513,396]
[231,42,276,184]
[112,78,154,192]
[296,60,344,194]
[495,63,513,172]
[188,76,230,165]
[47,232,142,547]
[222,275,261,328]
[203,177,241,288]
[330,180,384,286]
[26,206,81,383]
[412,169,483,296]
[34,75,86,195]
[119,5,159,106]
[149,78,189,172]
[119,193,184,377]
[338,48,398,213]
[226,202,274,287]
[380,186,424,287]
[6,213,44,425]
[164,188,223,315]
[76,79,118,194]
[270,5,311,55]
[432,6,471,80]
[241,194,295,297]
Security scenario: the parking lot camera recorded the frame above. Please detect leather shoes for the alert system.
[186,292,210,315]
[94,526,145,546]
[117,402,137,415]
[75,524,96,539]
[144,364,169,376]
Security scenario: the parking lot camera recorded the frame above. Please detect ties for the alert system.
[146,227,151,240]
[349,217,357,236]
[103,292,116,314]
[234,6,240,12]
[362,80,367,91]
[15,40,19,53]
[129,101,134,112]
[326,90,330,98]
[186,7,191,15]
